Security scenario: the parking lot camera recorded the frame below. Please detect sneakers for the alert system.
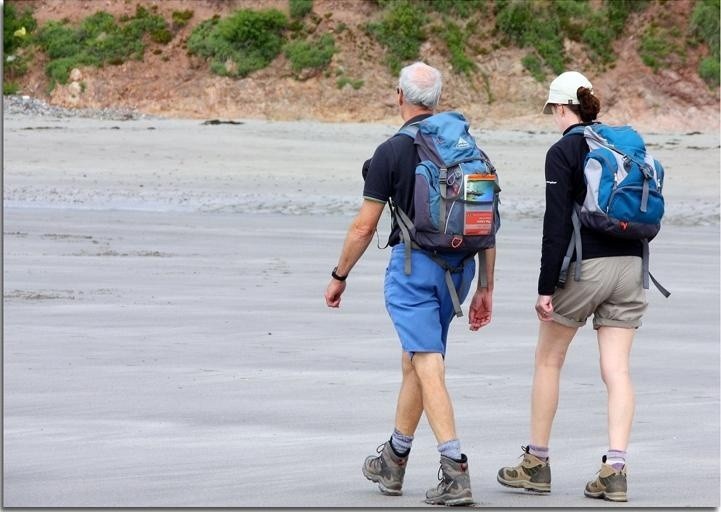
[498,448,552,495]
[584,455,630,501]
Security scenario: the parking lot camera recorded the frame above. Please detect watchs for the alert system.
[331,266,348,281]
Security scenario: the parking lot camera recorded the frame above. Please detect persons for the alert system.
[325,62,496,506]
[496,71,648,501]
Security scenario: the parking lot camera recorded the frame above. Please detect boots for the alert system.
[427,452,475,505]
[362,437,411,496]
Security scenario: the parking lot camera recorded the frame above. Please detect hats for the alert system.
[542,71,596,114]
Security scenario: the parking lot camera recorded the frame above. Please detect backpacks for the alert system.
[564,124,664,242]
[388,111,502,254]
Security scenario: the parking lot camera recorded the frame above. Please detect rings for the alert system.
[542,312,545,315]
